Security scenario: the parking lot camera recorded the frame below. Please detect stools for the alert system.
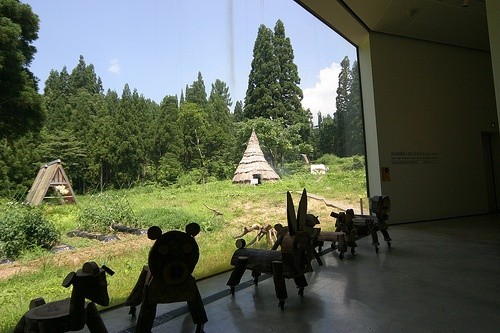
[12,186,395,333]
